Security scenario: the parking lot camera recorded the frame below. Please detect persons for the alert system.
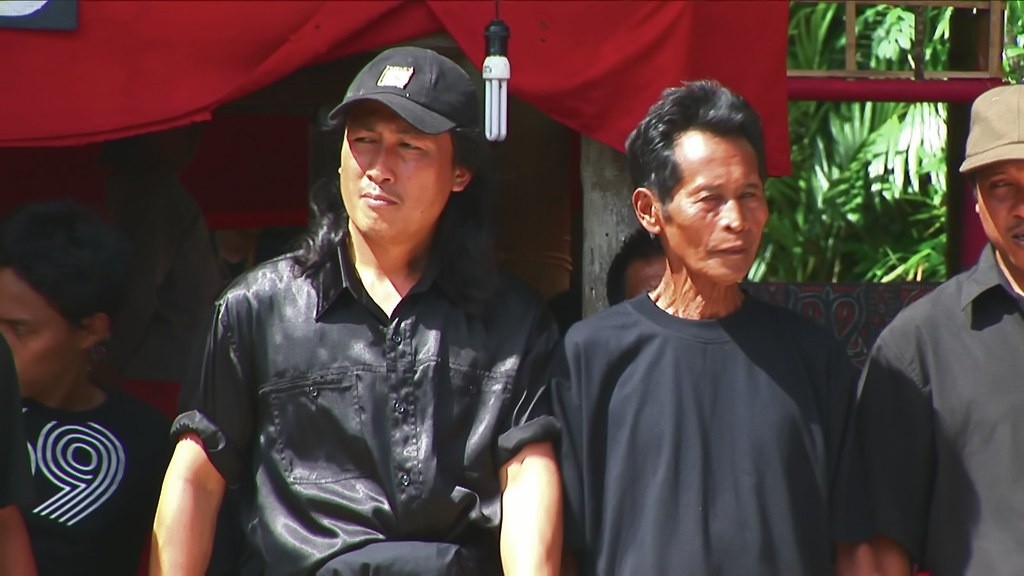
[552,80,851,575]
[151,47,563,576]
[0,199,234,576]
[606,227,667,307]
[836,82,1024,576]
[0,332,41,576]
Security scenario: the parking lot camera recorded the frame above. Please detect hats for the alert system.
[959,84,1024,172]
[328,46,482,135]
